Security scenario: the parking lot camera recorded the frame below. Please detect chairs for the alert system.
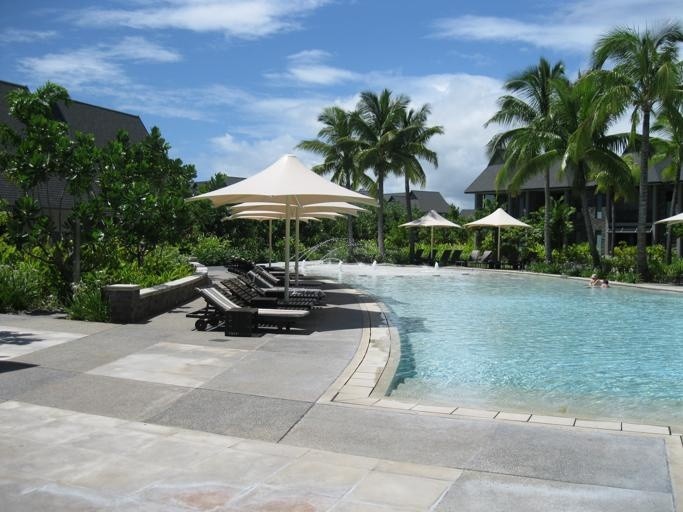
[413,248,492,268]
[186,256,325,333]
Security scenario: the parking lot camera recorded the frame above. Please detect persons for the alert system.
[590,273,609,288]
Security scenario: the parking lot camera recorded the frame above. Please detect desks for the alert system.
[225,308,258,337]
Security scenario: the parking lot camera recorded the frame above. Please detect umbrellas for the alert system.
[186,153,376,302]
[464,207,531,261]
[655,212,683,226]
[401,210,461,260]
